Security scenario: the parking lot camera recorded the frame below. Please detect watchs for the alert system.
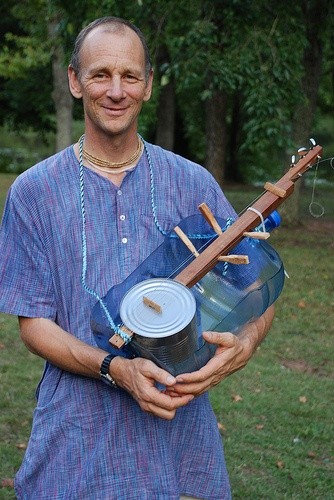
[100,355,121,389]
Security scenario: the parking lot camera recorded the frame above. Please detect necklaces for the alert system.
[80,134,145,175]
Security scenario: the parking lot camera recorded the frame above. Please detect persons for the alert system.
[1,17,276,500]
